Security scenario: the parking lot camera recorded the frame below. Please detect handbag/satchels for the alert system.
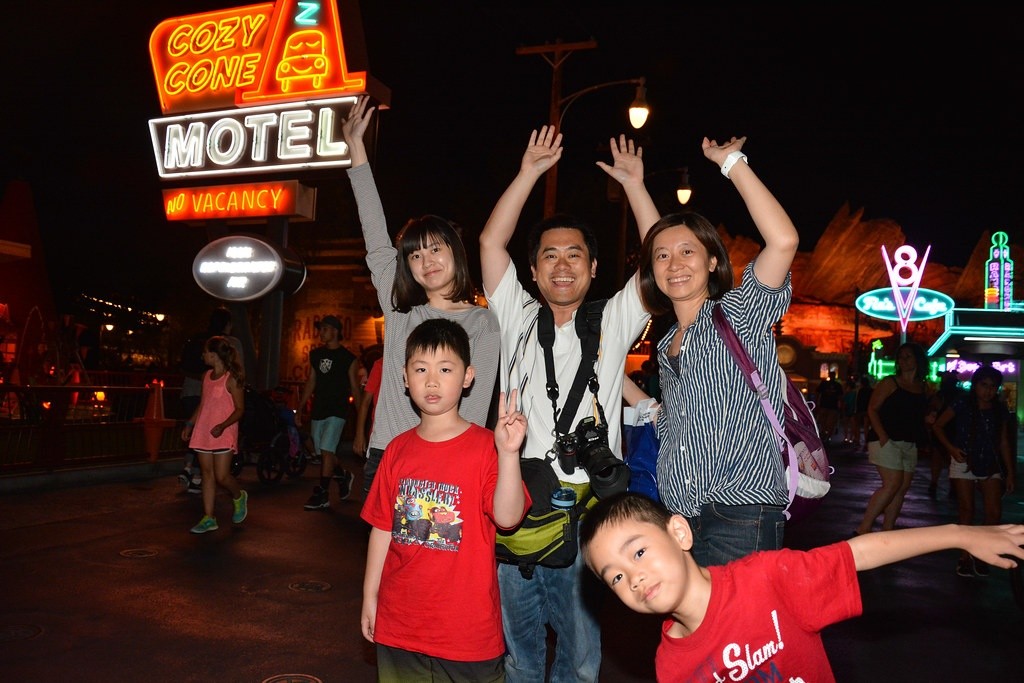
[496,455,598,578]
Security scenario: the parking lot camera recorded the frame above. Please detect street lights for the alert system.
[516,36,650,219]
[596,139,692,294]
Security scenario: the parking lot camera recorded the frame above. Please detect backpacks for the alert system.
[712,299,831,504]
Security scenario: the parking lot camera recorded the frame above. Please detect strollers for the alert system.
[230,384,309,484]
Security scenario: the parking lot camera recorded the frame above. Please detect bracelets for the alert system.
[721,151,747,179]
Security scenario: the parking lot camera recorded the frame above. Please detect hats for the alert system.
[312,315,343,340]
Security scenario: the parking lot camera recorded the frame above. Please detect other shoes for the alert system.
[188,480,202,494]
[178,470,190,489]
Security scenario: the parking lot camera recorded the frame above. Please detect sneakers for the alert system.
[190,515,219,533]
[304,486,331,508]
[232,490,248,523]
[955,559,991,579]
[332,469,354,506]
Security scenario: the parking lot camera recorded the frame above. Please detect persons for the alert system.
[579,492,1024,683]
[841,381,857,444]
[929,375,965,494]
[639,136,799,568]
[353,357,383,458]
[855,377,874,452]
[359,318,533,683]
[341,96,502,492]
[815,371,843,442]
[479,125,662,683]
[176,306,244,495]
[294,316,359,511]
[851,342,938,537]
[933,366,1016,577]
[181,336,250,532]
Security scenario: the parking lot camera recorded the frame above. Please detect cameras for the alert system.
[556,416,631,500]
